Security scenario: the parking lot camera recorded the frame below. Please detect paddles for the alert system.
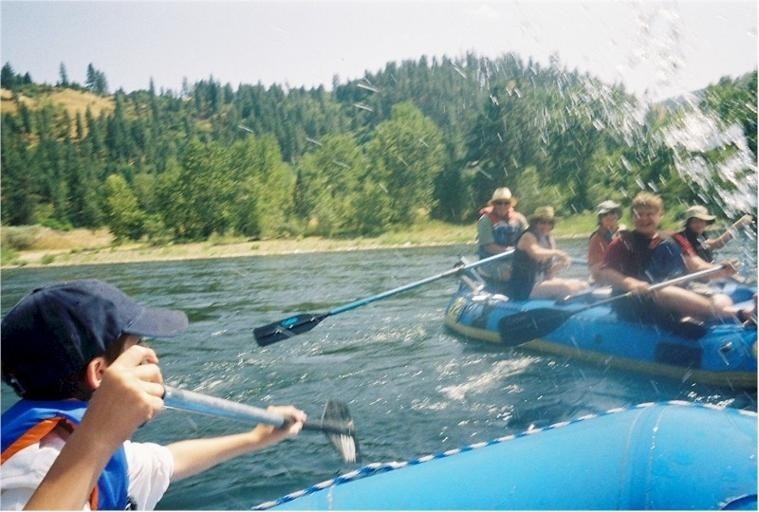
[252,249,515,347]
[138,383,361,464]
[497,265,728,347]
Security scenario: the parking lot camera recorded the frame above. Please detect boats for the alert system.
[445,257,756,394]
[235,395,758,508]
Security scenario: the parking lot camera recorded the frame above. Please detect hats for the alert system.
[683,206,716,224]
[487,187,516,207]
[1,279,189,395]
[527,207,557,223]
[597,201,623,222]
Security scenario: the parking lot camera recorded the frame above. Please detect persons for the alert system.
[0,279,309,510]
[586,189,757,332]
[588,200,628,282]
[470,186,531,286]
[506,205,589,304]
[666,202,753,285]
